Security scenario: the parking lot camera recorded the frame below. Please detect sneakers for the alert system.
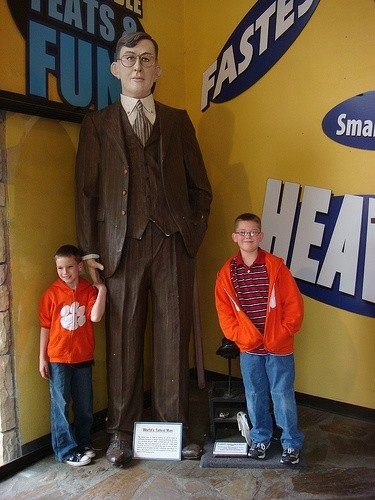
[248,440,272,459]
[279,447,299,464]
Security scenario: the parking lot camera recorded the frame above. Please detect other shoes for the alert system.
[65,452,91,467]
[82,446,96,457]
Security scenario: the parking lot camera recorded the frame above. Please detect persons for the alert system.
[39,245,107,466]
[215,213,303,464]
[76,32,213,465]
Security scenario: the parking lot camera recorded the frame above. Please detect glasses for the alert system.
[235,231,261,236]
